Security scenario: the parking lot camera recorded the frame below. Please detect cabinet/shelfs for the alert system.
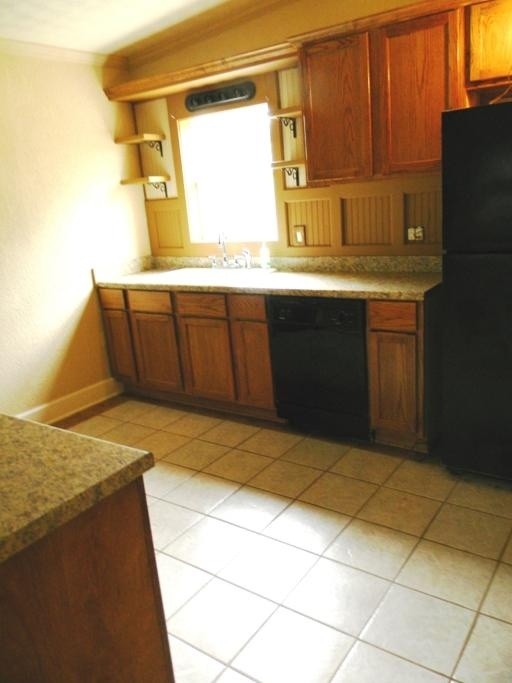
[364,299,431,454]
[267,106,307,186]
[464,0,512,95]
[296,7,464,188]
[115,96,179,201]
[98,288,290,427]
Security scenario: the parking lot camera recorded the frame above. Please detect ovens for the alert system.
[266,296,369,444]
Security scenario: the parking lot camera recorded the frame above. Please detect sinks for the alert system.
[164,267,277,284]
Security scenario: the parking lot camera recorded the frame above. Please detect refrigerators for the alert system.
[439,100,512,483]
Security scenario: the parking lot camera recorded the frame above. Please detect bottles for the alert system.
[259,242,271,270]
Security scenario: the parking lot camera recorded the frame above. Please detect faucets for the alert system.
[240,248,252,270]
[217,231,229,268]
